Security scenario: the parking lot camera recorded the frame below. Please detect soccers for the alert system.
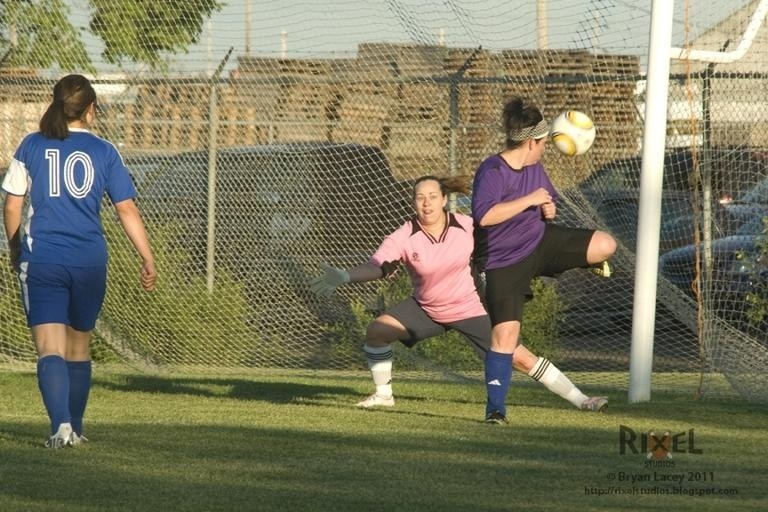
[551,110,595,156]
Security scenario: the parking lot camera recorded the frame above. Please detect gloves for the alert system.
[308,261,349,297]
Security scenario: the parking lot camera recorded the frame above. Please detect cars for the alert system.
[132,143,412,291]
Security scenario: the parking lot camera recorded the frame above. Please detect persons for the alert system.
[308,175,610,415]
[467,95,617,426]
[0,72,157,450]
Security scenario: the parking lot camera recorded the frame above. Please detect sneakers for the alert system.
[593,259,615,278]
[354,394,395,408]
[484,412,510,426]
[580,397,609,413]
[44,422,90,449]
